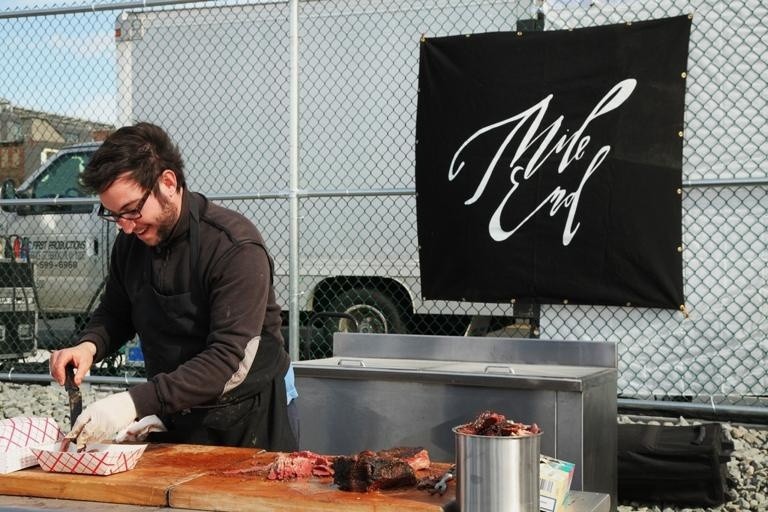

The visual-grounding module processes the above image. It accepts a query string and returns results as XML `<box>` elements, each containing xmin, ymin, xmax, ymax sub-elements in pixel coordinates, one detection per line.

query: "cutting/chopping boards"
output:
<box><xmin>168</xmin><ymin>449</ymin><xmax>458</xmax><ymax>512</ymax></box>
<box><xmin>1</xmin><ymin>439</ymin><xmax>269</xmax><ymax>507</ymax></box>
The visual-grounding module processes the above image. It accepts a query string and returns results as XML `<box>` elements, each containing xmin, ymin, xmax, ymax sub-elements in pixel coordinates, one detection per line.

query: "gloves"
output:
<box><xmin>63</xmin><ymin>390</ymin><xmax>139</xmax><ymax>453</ymax></box>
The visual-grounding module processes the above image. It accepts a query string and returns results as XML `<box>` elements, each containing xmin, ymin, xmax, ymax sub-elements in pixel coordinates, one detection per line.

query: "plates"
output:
<box><xmin>29</xmin><ymin>445</ymin><xmax>148</xmax><ymax>476</ymax></box>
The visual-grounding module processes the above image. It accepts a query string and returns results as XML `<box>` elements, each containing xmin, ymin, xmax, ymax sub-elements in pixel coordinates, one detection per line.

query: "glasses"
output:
<box><xmin>97</xmin><ymin>180</ymin><xmax>155</xmax><ymax>222</ymax></box>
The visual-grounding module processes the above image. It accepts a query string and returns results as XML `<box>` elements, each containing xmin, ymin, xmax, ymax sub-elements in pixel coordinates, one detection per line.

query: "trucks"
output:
<box><xmin>0</xmin><ymin>0</ymin><xmax>517</xmax><ymax>356</ymax></box>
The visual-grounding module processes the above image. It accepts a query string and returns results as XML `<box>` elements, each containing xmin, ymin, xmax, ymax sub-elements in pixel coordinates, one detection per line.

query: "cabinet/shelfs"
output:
<box><xmin>292</xmin><ymin>331</ymin><xmax>619</xmax><ymax>511</ymax></box>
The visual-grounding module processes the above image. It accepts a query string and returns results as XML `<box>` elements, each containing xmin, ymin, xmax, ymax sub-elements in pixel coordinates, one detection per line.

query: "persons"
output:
<box><xmin>48</xmin><ymin>123</ymin><xmax>300</xmax><ymax>453</ymax></box>
